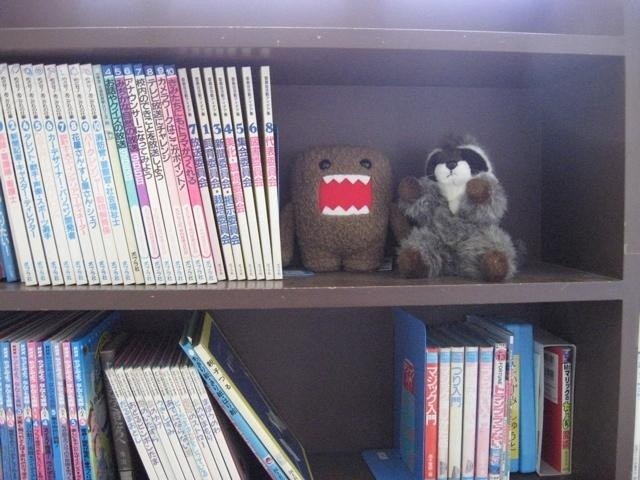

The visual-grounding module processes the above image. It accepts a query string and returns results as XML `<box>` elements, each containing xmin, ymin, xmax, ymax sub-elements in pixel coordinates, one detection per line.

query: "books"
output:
<box><xmin>401</xmin><ymin>314</ymin><xmax>577</xmax><ymax>480</ymax></box>
<box><xmin>1</xmin><ymin>311</ymin><xmax>313</xmax><ymax>480</ymax></box>
<box><xmin>1</xmin><ymin>62</ymin><xmax>283</xmax><ymax>289</ymax></box>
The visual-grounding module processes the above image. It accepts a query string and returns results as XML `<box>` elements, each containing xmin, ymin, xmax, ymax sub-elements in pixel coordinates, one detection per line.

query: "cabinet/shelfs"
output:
<box><xmin>0</xmin><ymin>0</ymin><xmax>640</xmax><ymax>480</ymax></box>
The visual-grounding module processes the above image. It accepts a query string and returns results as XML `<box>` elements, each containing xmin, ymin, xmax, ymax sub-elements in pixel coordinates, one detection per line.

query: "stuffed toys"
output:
<box><xmin>280</xmin><ymin>144</ymin><xmax>391</xmax><ymax>273</ymax></box>
<box><xmin>390</xmin><ymin>132</ymin><xmax>520</xmax><ymax>283</ymax></box>
